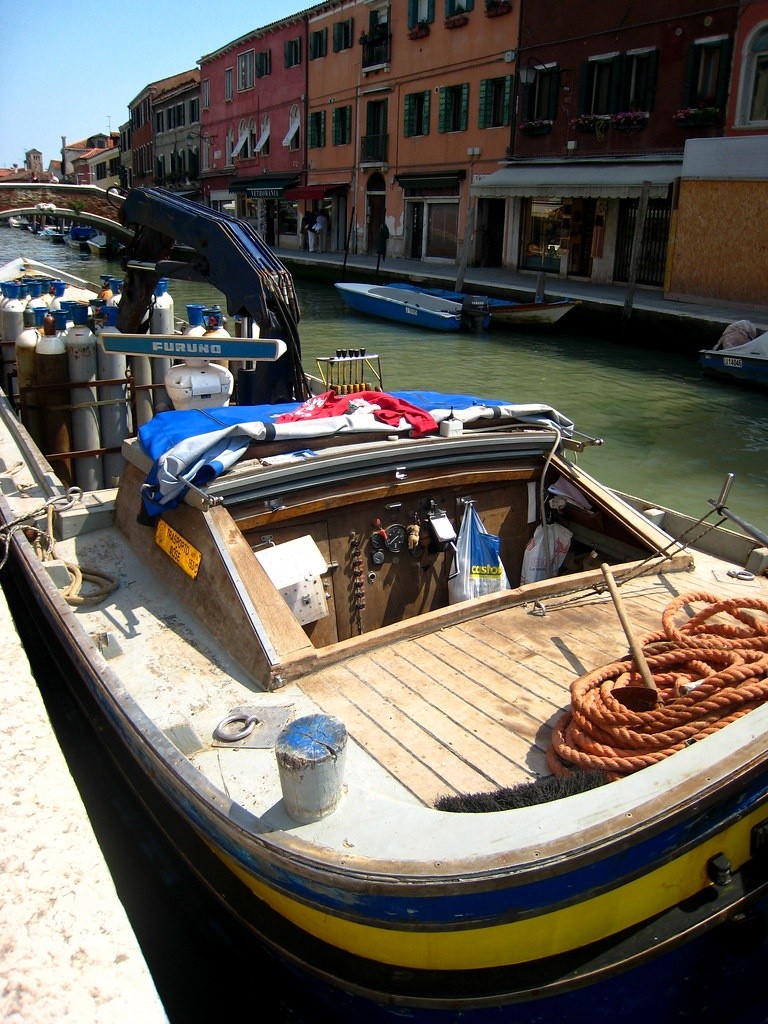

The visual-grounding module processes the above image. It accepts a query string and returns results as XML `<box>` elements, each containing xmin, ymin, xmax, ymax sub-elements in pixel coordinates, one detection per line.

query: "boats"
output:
<box><xmin>7</xmin><ymin>215</ymin><xmax>125</xmax><ymax>256</ymax></box>
<box><xmin>333</xmin><ymin>280</ymin><xmax>493</xmax><ymax>334</ymax></box>
<box><xmin>386</xmin><ymin>282</ymin><xmax>583</xmax><ymax>335</ymax></box>
<box><xmin>698</xmin><ymin>321</ymin><xmax>767</xmax><ymax>388</ymax></box>
<box><xmin>0</xmin><ymin>184</ymin><xmax>768</xmax><ymax>1024</ymax></box>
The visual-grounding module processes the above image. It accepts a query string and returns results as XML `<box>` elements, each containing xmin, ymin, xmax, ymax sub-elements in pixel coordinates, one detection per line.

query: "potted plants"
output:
<box><xmin>408</xmin><ymin>29</ymin><xmax>428</xmax><ymax>40</ymax></box>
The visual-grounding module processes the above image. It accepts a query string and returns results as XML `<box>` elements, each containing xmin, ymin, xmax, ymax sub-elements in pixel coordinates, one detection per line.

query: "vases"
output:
<box><xmin>485</xmin><ymin>7</ymin><xmax>511</xmax><ymax>17</ymax></box>
<box><xmin>443</xmin><ymin>17</ymin><xmax>470</xmax><ymax>29</ymax></box>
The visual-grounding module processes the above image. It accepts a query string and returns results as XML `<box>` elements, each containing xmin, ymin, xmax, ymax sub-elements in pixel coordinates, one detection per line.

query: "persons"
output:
<box><xmin>371</xmin><ymin>220</ymin><xmax>389</xmax><ymax>263</ymax></box>
<box><xmin>29</xmin><ymin>172</ymin><xmax>41</xmax><ymax>183</ymax></box>
<box><xmin>47</xmin><ymin>172</ymin><xmax>60</xmax><ymax>184</ymax></box>
<box><xmin>300</xmin><ymin>208</ymin><xmax>328</xmax><ymax>253</ymax></box>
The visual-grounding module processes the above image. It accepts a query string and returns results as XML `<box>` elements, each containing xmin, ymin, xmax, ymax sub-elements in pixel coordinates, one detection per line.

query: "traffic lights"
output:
<box><xmin>119</xmin><ymin>167</ymin><xmax>123</xmax><ymax>180</ymax></box>
<box><xmin>124</xmin><ymin>167</ymin><xmax>128</xmax><ymax>180</ymax></box>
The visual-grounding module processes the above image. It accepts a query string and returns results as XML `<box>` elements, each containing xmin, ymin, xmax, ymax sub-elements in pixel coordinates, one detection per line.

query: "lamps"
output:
<box><xmin>186</xmin><ymin>132</ymin><xmax>218</xmax><ymax>147</ymax></box>
<box><xmin>567</xmin><ymin>140</ymin><xmax>577</xmax><ymax>156</ymax></box>
<box><xmin>467</xmin><ymin>147</ymin><xmax>481</xmax><ymax>162</ymax></box>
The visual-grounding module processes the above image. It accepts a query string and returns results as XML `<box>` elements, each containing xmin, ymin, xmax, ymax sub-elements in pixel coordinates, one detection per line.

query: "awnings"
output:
<box><xmin>285</xmin><ymin>183</ymin><xmax>350</xmax><ymax>200</ymax></box>
<box><xmin>228</xmin><ymin>172</ymin><xmax>300</xmax><ymax>199</ymax></box>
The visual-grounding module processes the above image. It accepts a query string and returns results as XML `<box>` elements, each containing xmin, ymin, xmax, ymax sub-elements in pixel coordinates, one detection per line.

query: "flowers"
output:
<box><xmin>484</xmin><ymin>0</ymin><xmax>512</xmax><ymax>11</ymax></box>
<box><xmin>412</xmin><ymin>17</ymin><xmax>428</xmax><ymax>33</ymax></box>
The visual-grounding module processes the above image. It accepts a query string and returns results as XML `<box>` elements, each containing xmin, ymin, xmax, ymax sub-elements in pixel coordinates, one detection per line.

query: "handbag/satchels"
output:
<box><xmin>314</xmin><ymin>223</ymin><xmax>323</xmax><ymax>234</ymax></box>
<box><xmin>520</xmin><ymin>522</ymin><xmax>574</xmax><ymax>586</ymax></box>
<box><xmin>304</xmin><ymin>224</ymin><xmax>309</xmax><ymax>230</ymax></box>
<box><xmin>448</xmin><ymin>502</ymin><xmax>511</xmax><ymax>605</ymax></box>
